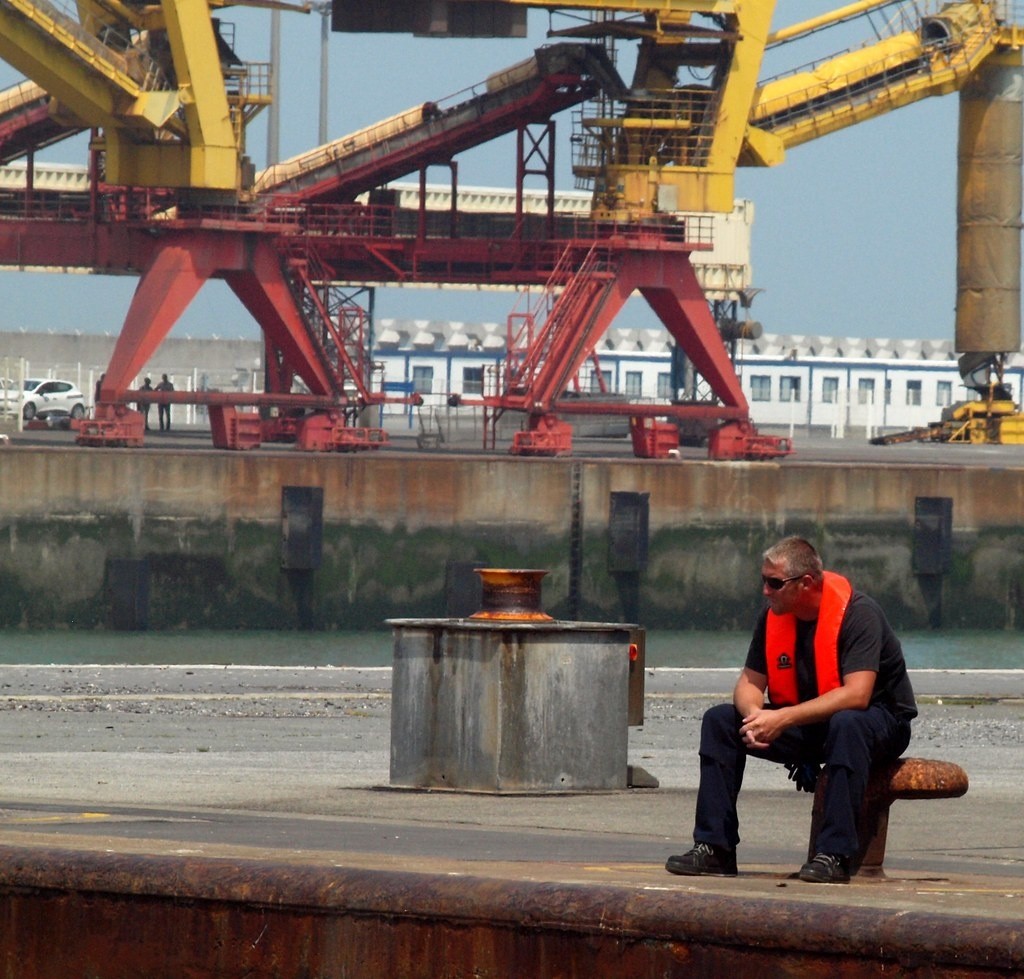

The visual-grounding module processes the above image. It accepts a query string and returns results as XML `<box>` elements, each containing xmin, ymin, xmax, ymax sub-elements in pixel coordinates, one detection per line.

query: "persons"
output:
<box><xmin>154</xmin><ymin>373</ymin><xmax>174</xmax><ymax>432</ymax></box>
<box><xmin>137</xmin><ymin>377</ymin><xmax>153</xmax><ymax>430</ymax></box>
<box><xmin>94</xmin><ymin>373</ymin><xmax>105</xmax><ymax>402</ymax></box>
<box><xmin>664</xmin><ymin>534</ymin><xmax>918</xmax><ymax>884</ymax></box>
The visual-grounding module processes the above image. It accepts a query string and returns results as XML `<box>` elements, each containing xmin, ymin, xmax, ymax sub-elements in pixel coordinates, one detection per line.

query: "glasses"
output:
<box><xmin>761</xmin><ymin>574</ymin><xmax>804</xmax><ymax>590</ymax></box>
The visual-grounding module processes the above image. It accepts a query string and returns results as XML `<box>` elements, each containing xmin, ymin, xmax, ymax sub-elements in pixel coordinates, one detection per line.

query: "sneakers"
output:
<box><xmin>665</xmin><ymin>842</ymin><xmax>738</xmax><ymax>877</ymax></box>
<box><xmin>799</xmin><ymin>852</ymin><xmax>851</xmax><ymax>884</ymax></box>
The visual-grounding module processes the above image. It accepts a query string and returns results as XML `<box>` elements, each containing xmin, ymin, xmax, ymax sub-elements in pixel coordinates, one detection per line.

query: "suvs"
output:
<box><xmin>0</xmin><ymin>378</ymin><xmax>89</xmax><ymax>422</ymax></box>
